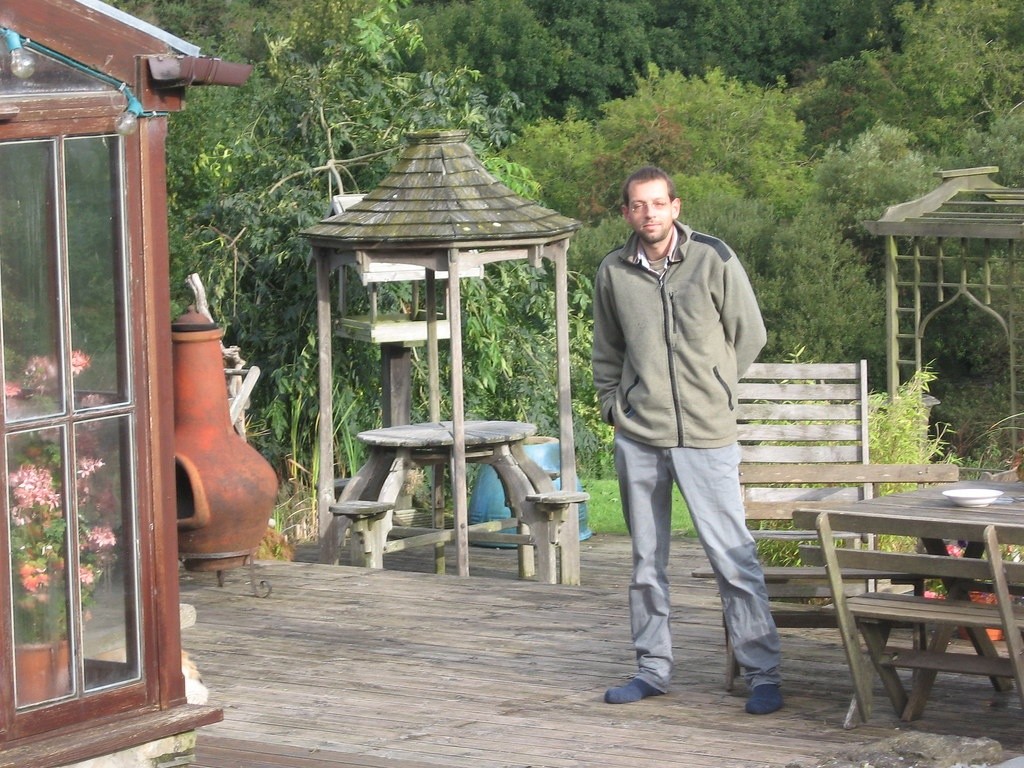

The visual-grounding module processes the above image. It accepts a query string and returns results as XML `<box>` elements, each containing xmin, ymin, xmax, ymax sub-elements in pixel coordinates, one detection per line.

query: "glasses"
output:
<box><xmin>629</xmin><ymin>199</ymin><xmax>669</xmax><ymax>213</ymax></box>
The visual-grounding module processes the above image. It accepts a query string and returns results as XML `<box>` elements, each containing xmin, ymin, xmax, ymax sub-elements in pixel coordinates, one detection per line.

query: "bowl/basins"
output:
<box><xmin>466</xmin><ymin>435</ymin><xmax>593</xmax><ymax>548</ymax></box>
<box><xmin>942</xmin><ymin>489</ymin><xmax>1005</xmax><ymax>508</ymax></box>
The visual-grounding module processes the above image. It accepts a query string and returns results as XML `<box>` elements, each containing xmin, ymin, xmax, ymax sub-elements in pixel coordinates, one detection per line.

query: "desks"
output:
<box><xmin>790</xmin><ymin>479</ymin><xmax>1024</xmax><ymax>722</ymax></box>
<box><xmin>338</xmin><ymin>420</ymin><xmax>557</xmax><ymax>577</ymax></box>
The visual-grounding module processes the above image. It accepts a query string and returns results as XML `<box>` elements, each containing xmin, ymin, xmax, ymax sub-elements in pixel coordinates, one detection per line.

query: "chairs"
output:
<box><xmin>690</xmin><ymin>359</ymin><xmax>1024</xmax><ymax>731</ymax></box>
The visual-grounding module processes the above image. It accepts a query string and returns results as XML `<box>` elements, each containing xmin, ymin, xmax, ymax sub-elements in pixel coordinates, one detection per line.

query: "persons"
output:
<box><xmin>592</xmin><ymin>164</ymin><xmax>785</xmax><ymax>716</ymax></box>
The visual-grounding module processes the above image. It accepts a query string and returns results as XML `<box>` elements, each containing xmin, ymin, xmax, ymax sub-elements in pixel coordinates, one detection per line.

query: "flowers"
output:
<box><xmin>5</xmin><ymin>340</ymin><xmax>122</xmax><ymax>644</ymax></box>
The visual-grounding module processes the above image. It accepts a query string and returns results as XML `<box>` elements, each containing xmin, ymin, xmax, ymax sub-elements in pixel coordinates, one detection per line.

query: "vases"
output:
<box><xmin>13</xmin><ymin>638</ymin><xmax>71</xmax><ymax>709</ymax></box>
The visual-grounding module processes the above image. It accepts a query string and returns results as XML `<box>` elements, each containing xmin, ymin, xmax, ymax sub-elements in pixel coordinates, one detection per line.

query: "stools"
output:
<box><xmin>309</xmin><ymin>469</ymin><xmax>591</xmax><ymax>585</ymax></box>
